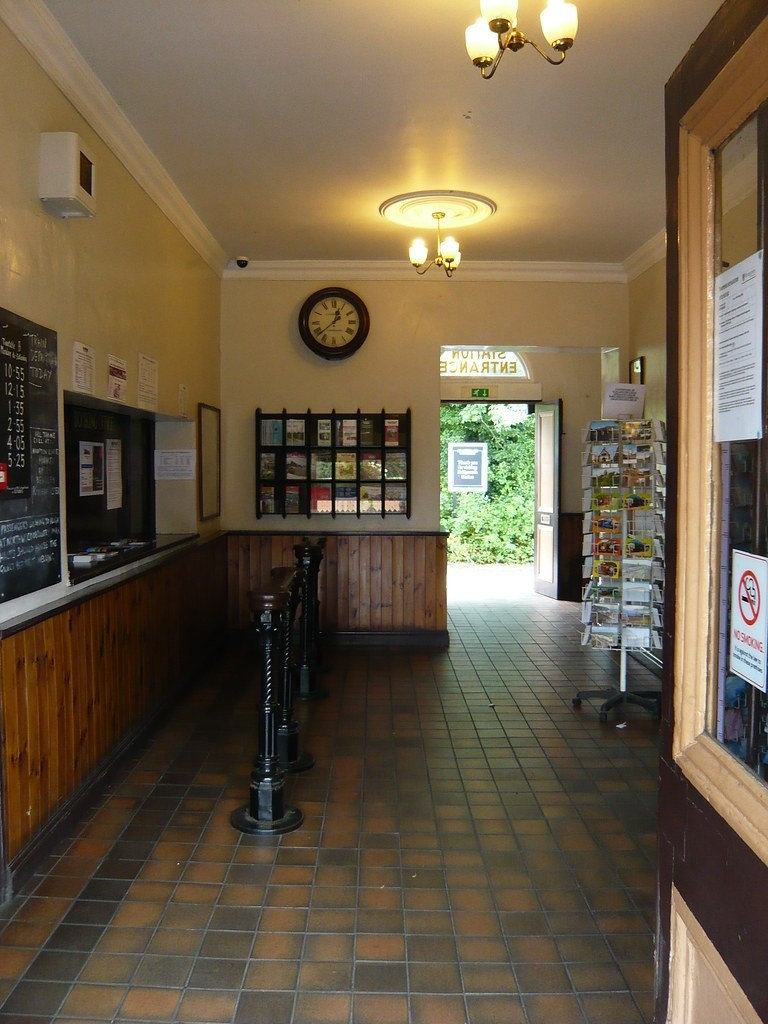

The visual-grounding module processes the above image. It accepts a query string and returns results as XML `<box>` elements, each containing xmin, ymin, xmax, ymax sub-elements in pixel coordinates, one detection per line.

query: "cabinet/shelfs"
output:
<box><xmin>254</xmin><ymin>407</ymin><xmax>412</xmax><ymax>520</ymax></box>
<box><xmin>572</xmin><ymin>413</ymin><xmax>667</xmax><ymax>722</ymax></box>
<box><xmin>722</xmin><ymin>443</ymin><xmax>768</xmax><ymax>781</ymax></box>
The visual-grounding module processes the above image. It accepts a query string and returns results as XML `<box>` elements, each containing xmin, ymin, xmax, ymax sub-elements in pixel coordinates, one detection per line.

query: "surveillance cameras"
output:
<box><xmin>237</xmin><ymin>256</ymin><xmax>249</xmax><ymax>268</ymax></box>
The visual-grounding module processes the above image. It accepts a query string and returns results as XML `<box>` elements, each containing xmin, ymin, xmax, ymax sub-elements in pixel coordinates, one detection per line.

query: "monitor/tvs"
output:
<box><xmin>62</xmin><ymin>389</ymin><xmax>158</xmax><ymax>555</ymax></box>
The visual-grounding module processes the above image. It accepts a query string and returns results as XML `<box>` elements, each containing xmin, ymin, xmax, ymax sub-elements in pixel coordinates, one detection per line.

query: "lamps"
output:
<box><xmin>465</xmin><ymin>0</ymin><xmax>579</xmax><ymax>80</ymax></box>
<box><xmin>379</xmin><ymin>191</ymin><xmax>496</xmax><ymax>278</ymax></box>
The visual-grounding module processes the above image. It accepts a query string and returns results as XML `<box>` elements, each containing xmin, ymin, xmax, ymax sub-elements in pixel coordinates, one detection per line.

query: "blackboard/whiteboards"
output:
<box><xmin>0</xmin><ymin>306</ymin><xmax>62</xmax><ymax>602</ymax></box>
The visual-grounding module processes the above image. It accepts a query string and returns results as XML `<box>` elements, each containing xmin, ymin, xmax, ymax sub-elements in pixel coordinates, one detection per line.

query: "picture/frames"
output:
<box><xmin>628</xmin><ymin>356</ymin><xmax>643</xmax><ymax>388</ymax></box>
<box><xmin>198</xmin><ymin>402</ymin><xmax>221</xmax><ymax>520</ymax></box>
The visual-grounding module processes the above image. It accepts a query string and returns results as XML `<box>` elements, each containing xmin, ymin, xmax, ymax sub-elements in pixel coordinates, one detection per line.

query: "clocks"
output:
<box><xmin>299</xmin><ymin>287</ymin><xmax>370</xmax><ymax>361</ymax></box>
